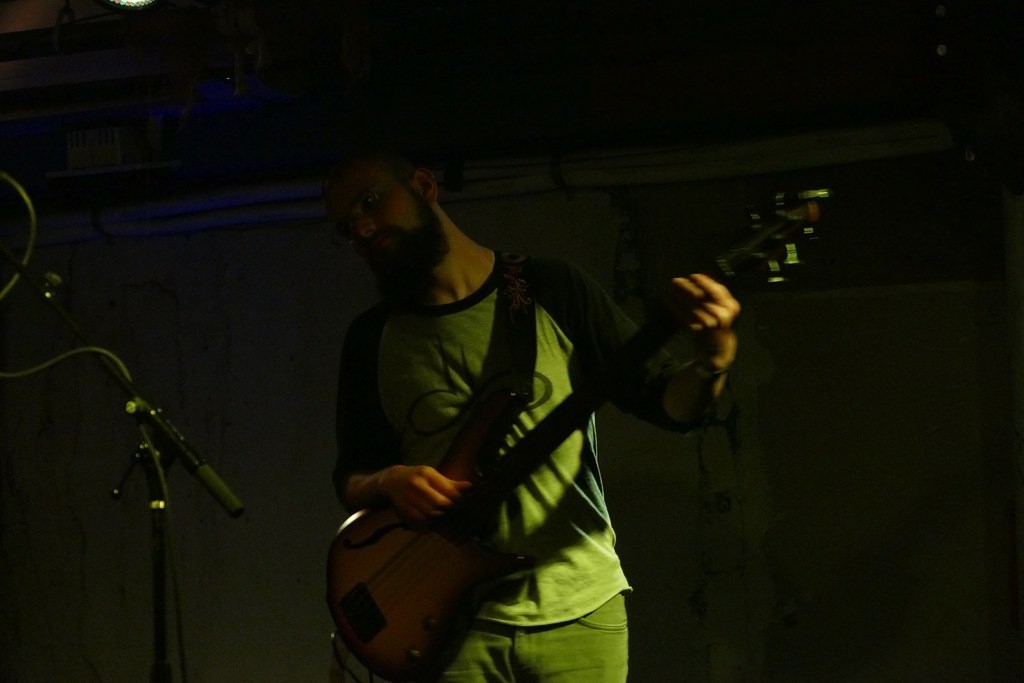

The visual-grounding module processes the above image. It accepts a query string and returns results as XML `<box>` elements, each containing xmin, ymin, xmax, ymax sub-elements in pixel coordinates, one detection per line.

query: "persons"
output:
<box><xmin>321</xmin><ymin>151</ymin><xmax>746</xmax><ymax>683</ymax></box>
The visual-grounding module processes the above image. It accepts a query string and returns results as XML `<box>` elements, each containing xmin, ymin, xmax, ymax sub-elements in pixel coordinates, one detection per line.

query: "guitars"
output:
<box><xmin>326</xmin><ymin>188</ymin><xmax>832</xmax><ymax>683</ymax></box>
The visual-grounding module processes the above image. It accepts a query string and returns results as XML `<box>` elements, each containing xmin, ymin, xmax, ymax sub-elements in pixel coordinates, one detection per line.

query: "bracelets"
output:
<box><xmin>694</xmin><ymin>365</ymin><xmax>729</xmax><ymax>381</ymax></box>
